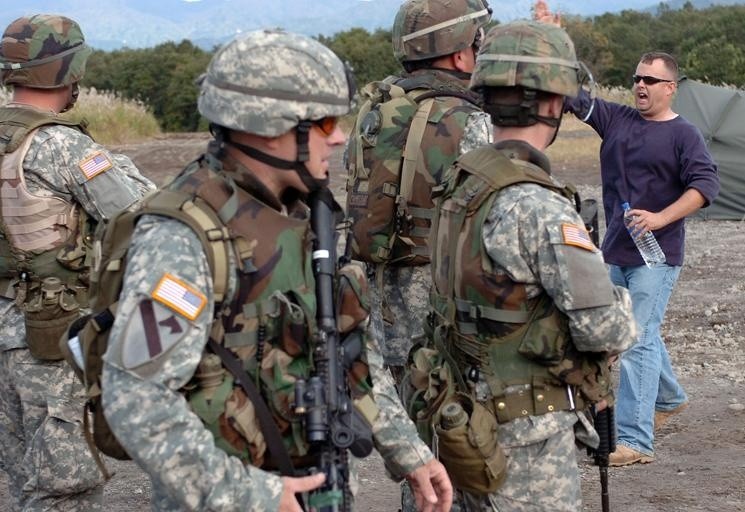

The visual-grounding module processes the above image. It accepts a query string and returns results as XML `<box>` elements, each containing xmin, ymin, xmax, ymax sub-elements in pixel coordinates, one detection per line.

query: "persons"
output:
<box><xmin>0</xmin><ymin>12</ymin><xmax>163</xmax><ymax>511</ymax></box>
<box><xmin>340</xmin><ymin>0</ymin><xmax>495</xmax><ymax>410</ymax></box>
<box><xmin>529</xmin><ymin>1</ymin><xmax>722</xmax><ymax>467</ymax></box>
<box><xmin>426</xmin><ymin>16</ymin><xmax>639</xmax><ymax>511</ymax></box>
<box><xmin>98</xmin><ymin>28</ymin><xmax>452</xmax><ymax>510</ymax></box>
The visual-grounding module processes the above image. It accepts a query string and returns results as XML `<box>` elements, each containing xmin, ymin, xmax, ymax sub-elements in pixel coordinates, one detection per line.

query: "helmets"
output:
<box><xmin>0</xmin><ymin>12</ymin><xmax>87</xmax><ymax>88</ymax></box>
<box><xmin>199</xmin><ymin>30</ymin><xmax>353</xmax><ymax>140</ymax></box>
<box><xmin>468</xmin><ymin>20</ymin><xmax>578</xmax><ymax>100</ymax></box>
<box><xmin>391</xmin><ymin>0</ymin><xmax>492</xmax><ymax>63</ymax></box>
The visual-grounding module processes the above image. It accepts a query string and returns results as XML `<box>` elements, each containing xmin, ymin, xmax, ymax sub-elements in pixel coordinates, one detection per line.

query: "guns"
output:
<box><xmin>293</xmin><ymin>189</ymin><xmax>372</xmax><ymax>512</ymax></box>
<box><xmin>560</xmin><ymin>200</ymin><xmax>617</xmax><ymax>511</ymax></box>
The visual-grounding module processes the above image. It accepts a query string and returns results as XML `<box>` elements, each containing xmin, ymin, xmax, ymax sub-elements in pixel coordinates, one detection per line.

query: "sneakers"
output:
<box><xmin>655</xmin><ymin>400</ymin><xmax>688</xmax><ymax>429</ymax></box>
<box><xmin>609</xmin><ymin>443</ymin><xmax>653</xmax><ymax>466</ymax></box>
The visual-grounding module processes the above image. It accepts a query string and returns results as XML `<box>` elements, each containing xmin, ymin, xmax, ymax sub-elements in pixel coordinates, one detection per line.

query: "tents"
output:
<box><xmin>669</xmin><ymin>72</ymin><xmax>745</xmax><ymax>223</ymax></box>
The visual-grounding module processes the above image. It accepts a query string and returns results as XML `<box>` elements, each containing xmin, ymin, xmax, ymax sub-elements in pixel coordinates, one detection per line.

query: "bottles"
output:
<box><xmin>440</xmin><ymin>404</ymin><xmax>505</xmax><ymax>496</ymax></box>
<box><xmin>621</xmin><ymin>203</ymin><xmax>667</xmax><ymax>269</ymax></box>
<box><xmin>24</xmin><ymin>277</ymin><xmax>82</xmax><ymax>361</ymax></box>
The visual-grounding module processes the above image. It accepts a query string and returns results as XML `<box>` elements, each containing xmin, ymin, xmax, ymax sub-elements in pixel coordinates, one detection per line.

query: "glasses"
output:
<box><xmin>632</xmin><ymin>73</ymin><xmax>671</xmax><ymax>87</ymax></box>
<box><xmin>314</xmin><ymin>113</ymin><xmax>339</xmax><ymax>135</ymax></box>
